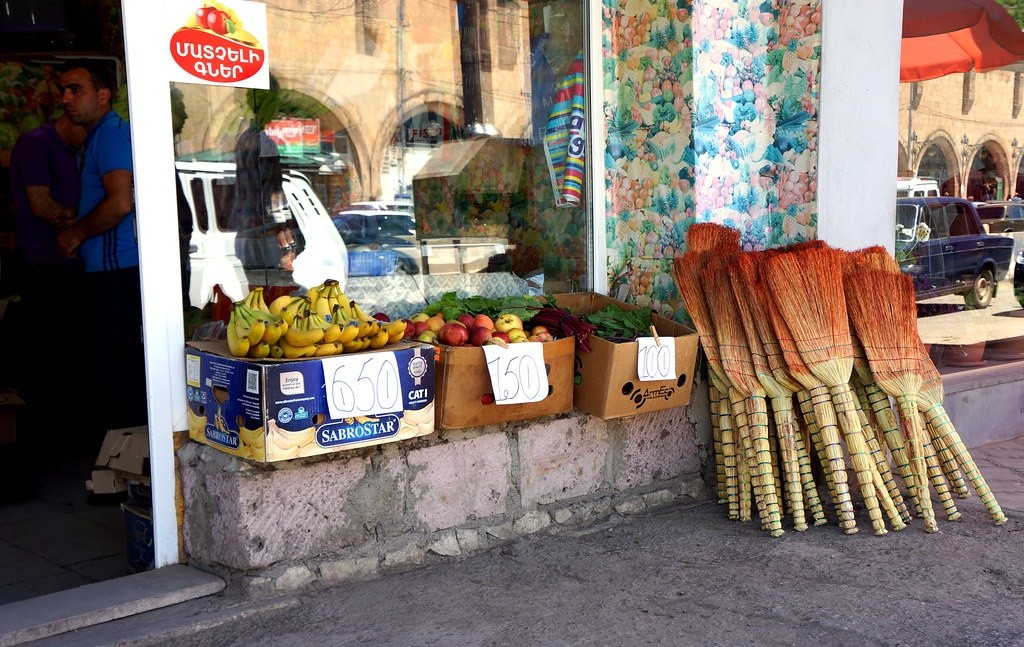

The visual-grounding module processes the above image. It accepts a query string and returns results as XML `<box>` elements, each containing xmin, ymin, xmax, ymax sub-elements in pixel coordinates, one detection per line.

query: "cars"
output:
<box><xmin>332</xmin><ymin>202</ymin><xmax>420</xmax><ymax>277</ymax></box>
<box><xmin>176</xmin><ymin>159</ymin><xmax>348</xmax><ymax>312</ymax></box>
<box><xmin>895</xmin><ymin>198</ymin><xmax>1014</xmax><ymax>309</ymax></box>
<box><xmin>896</xmin><ymin>178</ymin><xmax>1024</xmax><ymax>233</ymax></box>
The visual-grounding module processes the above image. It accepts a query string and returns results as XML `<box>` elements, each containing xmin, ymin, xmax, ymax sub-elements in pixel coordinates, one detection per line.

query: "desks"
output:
<box><xmin>343</xmin><ymin>272</ymin><xmax>530</xmax><ymax>324</ymax></box>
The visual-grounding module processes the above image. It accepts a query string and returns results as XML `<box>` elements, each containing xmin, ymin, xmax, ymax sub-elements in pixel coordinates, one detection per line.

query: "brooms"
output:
<box><xmin>668</xmin><ymin>222</ymin><xmax>1010</xmax><ymax>539</ymax></box>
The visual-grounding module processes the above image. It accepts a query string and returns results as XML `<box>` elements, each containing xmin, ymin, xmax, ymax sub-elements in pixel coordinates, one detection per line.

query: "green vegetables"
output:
<box><xmin>423</xmin><ymin>290</ymin><xmax>658</xmax><ymax>353</ymax></box>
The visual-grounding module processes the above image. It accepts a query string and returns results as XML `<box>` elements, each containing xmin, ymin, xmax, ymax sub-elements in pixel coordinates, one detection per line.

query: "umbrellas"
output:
<box><xmin>899</xmin><ymin>0</ymin><xmax>1024</xmax><ymax>86</ymax></box>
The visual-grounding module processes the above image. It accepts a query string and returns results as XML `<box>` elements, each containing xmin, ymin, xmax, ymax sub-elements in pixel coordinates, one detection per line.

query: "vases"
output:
<box><xmin>923</xmin><ymin>309</ymin><xmax>1024</xmax><ymax>361</ymax></box>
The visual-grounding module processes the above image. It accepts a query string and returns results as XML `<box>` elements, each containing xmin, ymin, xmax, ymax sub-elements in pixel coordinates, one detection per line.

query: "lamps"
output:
<box><xmin>911</xmin><ymin>130</ymin><xmax>937</xmax><ymax>157</ymax></box>
<box><xmin>961</xmin><ymin>135</ymin><xmax>987</xmax><ymax>162</ymax></box>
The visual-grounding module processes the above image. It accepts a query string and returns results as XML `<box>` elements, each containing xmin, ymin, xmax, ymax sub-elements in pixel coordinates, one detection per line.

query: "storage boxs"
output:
<box><xmin>532</xmin><ymin>291</ymin><xmax>699</xmax><ymax>421</ymax></box>
<box><xmin>0</xmin><ymin>386</ymin><xmax>26</xmax><ymax>445</ymax></box>
<box><xmin>178</xmin><ymin>337</ymin><xmax>437</xmax><ymax>461</ymax></box>
<box><xmin>435</xmin><ymin>331</ymin><xmax>574</xmax><ymax>432</ymax></box>
<box><xmin>348</xmin><ymin>249</ymin><xmax>398</xmax><ymax>276</ymax></box>
<box><xmin>87</xmin><ymin>426</ymin><xmax>155</xmax><ymax>572</ymax></box>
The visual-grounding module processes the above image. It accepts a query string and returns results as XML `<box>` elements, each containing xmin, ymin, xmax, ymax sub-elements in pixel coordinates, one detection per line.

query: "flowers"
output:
<box><xmin>893</xmin><ymin>222</ymin><xmax>931</xmax><ymax>265</ymax></box>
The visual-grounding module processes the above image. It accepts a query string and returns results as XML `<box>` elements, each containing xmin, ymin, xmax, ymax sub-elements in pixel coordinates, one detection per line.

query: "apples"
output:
<box><xmin>371</xmin><ymin>312</ymin><xmax>553</xmax><ymax>346</ymax></box>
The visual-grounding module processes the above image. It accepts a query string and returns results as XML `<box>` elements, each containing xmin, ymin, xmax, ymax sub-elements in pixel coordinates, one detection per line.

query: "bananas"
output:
<box><xmin>227</xmin><ymin>278</ymin><xmax>407</xmax><ymax>358</ymax></box>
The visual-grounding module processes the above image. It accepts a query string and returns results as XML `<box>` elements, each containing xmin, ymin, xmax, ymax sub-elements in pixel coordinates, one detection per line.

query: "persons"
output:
<box><xmin>234</xmin><ymin>73</ymin><xmax>306</xmax><ymax>295</ymax></box>
<box><xmin>170</xmin><ymin>86</ymin><xmax>193</xmax><ymax>323</ymax></box>
<box><xmin>10</xmin><ymin>112</ymin><xmax>88</xmax><ymax>360</ymax></box>
<box><xmin>968</xmin><ymin>184</ymin><xmax>996</xmax><ymax>202</ymax></box>
<box><xmin>55</xmin><ymin>58</ymin><xmax>143</xmax><ymax>430</ymax></box>
<box><xmin>1012</xmin><ymin>193</ymin><xmax>1022</xmax><ymax>201</ymax></box>
